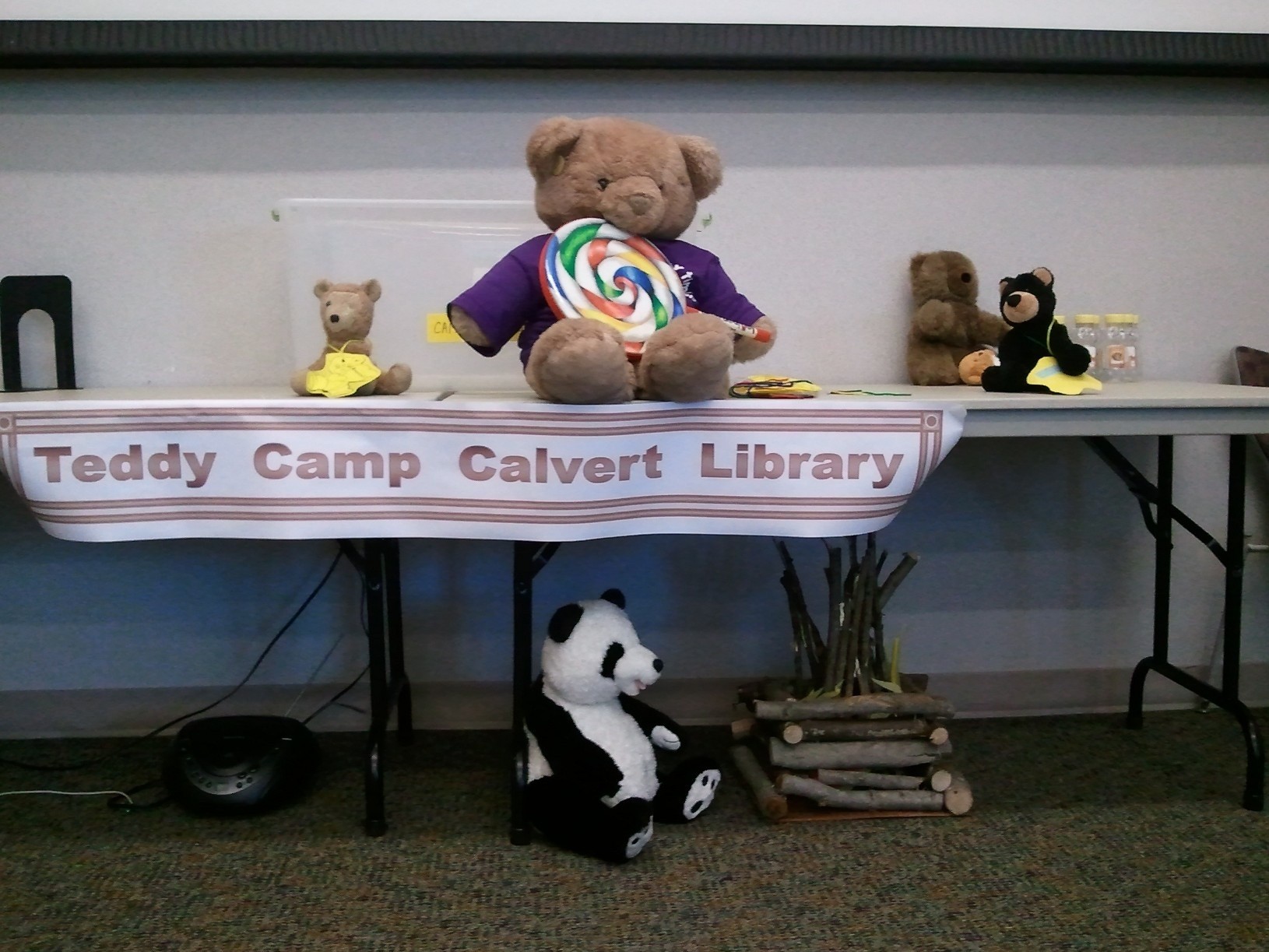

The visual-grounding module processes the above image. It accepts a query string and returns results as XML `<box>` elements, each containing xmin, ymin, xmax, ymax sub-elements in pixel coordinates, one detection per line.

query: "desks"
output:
<box><xmin>0</xmin><ymin>377</ymin><xmax>1269</xmax><ymax>835</ymax></box>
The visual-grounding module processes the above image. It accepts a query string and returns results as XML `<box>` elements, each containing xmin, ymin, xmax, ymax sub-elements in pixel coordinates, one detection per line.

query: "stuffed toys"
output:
<box><xmin>980</xmin><ymin>266</ymin><xmax>1092</xmax><ymax>393</ymax></box>
<box><xmin>445</xmin><ymin>116</ymin><xmax>777</xmax><ymax>405</ymax></box>
<box><xmin>289</xmin><ymin>278</ymin><xmax>411</xmax><ymax>399</ymax></box>
<box><xmin>905</xmin><ymin>251</ymin><xmax>1012</xmax><ymax>386</ymax></box>
<box><xmin>521</xmin><ymin>588</ymin><xmax>721</xmax><ymax>865</ymax></box>
<box><xmin>958</xmin><ymin>349</ymin><xmax>995</xmax><ymax>386</ymax></box>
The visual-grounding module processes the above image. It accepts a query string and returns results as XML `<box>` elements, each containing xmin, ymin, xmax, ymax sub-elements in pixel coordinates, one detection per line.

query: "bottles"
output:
<box><xmin>1095</xmin><ymin>314</ymin><xmax>1142</xmax><ymax>384</ymax></box>
<box><xmin>1072</xmin><ymin>314</ymin><xmax>1107</xmax><ymax>381</ymax></box>
<box><xmin>1054</xmin><ymin>315</ymin><xmax>1067</xmax><ymax>325</ymax></box>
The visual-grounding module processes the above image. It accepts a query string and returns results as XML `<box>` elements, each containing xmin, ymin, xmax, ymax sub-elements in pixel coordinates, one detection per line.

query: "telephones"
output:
<box><xmin>163</xmin><ymin>713</ymin><xmax>318</xmax><ymax>816</ymax></box>
